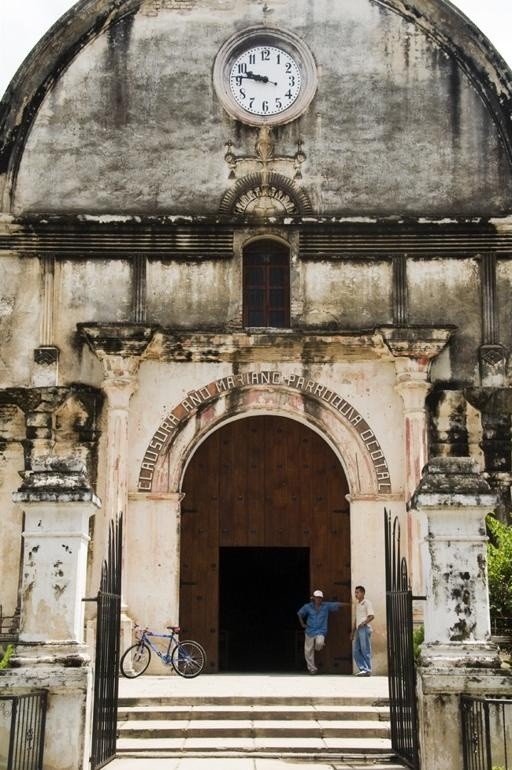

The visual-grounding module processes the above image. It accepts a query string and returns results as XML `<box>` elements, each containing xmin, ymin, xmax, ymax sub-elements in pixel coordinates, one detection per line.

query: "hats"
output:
<box><xmin>313</xmin><ymin>589</ymin><xmax>323</xmax><ymax>598</ymax></box>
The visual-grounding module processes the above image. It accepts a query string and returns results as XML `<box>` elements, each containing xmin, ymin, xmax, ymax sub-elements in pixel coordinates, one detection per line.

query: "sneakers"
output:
<box><xmin>310</xmin><ymin>670</ymin><xmax>317</xmax><ymax>676</ymax></box>
<box><xmin>355</xmin><ymin>671</ymin><xmax>372</xmax><ymax>677</ymax></box>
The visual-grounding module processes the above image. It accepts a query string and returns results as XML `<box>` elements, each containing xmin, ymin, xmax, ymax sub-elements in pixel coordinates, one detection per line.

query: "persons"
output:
<box><xmin>349</xmin><ymin>586</ymin><xmax>375</xmax><ymax>677</ymax></box>
<box><xmin>296</xmin><ymin>589</ymin><xmax>351</xmax><ymax>675</ymax></box>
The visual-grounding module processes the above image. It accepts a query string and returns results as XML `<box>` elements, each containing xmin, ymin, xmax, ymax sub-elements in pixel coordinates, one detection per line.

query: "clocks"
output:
<box><xmin>211</xmin><ymin>24</ymin><xmax>318</xmax><ymax>128</ymax></box>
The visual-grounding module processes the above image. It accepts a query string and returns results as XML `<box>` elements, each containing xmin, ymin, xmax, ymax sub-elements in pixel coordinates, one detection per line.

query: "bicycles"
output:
<box><xmin>119</xmin><ymin>620</ymin><xmax>208</xmax><ymax>680</ymax></box>
